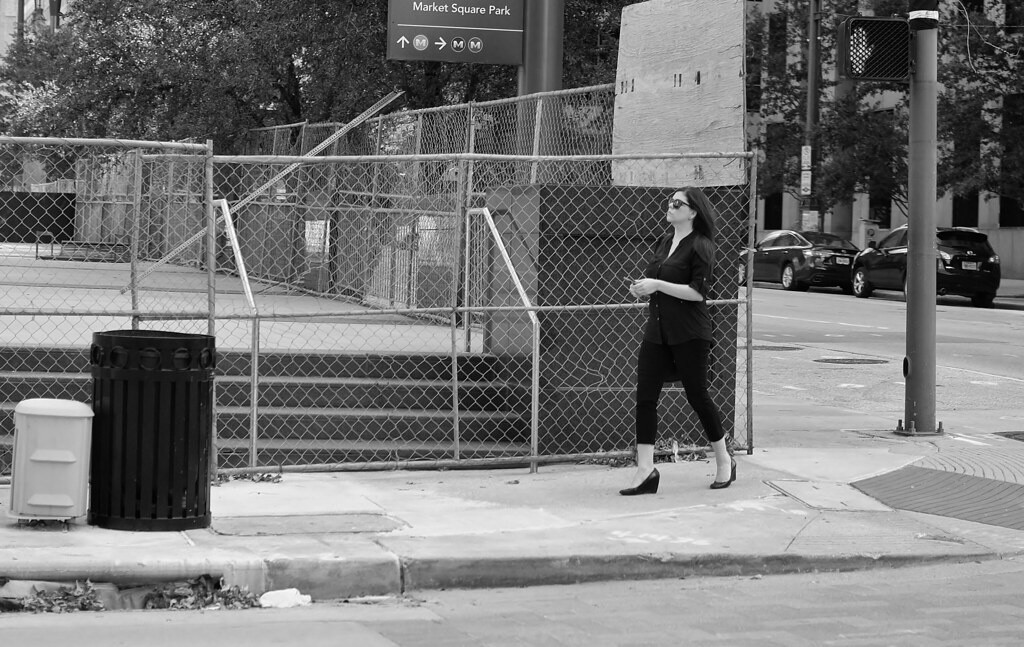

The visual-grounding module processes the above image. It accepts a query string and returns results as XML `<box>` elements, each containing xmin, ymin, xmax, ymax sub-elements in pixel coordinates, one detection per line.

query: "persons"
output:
<box><xmin>619</xmin><ymin>187</ymin><xmax>737</xmax><ymax>496</ymax></box>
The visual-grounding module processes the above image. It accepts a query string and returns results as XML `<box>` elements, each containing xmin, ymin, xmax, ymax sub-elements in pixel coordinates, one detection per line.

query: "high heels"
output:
<box><xmin>710</xmin><ymin>457</ymin><xmax>736</xmax><ymax>489</ymax></box>
<box><xmin>619</xmin><ymin>467</ymin><xmax>660</xmax><ymax>495</ymax></box>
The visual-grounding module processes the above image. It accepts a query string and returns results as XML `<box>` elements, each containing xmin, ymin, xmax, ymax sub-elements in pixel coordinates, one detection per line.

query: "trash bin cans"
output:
<box><xmin>6</xmin><ymin>397</ymin><xmax>94</xmax><ymax>526</ymax></box>
<box><xmin>88</xmin><ymin>329</ymin><xmax>218</xmax><ymax>534</ymax></box>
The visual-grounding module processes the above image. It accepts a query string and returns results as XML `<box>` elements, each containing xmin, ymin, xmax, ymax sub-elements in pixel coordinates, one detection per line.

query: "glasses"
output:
<box><xmin>669</xmin><ymin>197</ymin><xmax>689</xmax><ymax>209</ymax></box>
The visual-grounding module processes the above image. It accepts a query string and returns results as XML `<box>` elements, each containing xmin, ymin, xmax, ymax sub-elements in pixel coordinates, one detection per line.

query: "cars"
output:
<box><xmin>737</xmin><ymin>230</ymin><xmax>859</xmax><ymax>290</ymax></box>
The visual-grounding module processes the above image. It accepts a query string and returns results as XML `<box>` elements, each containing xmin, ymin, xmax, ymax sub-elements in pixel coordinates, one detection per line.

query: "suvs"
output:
<box><xmin>851</xmin><ymin>223</ymin><xmax>1001</xmax><ymax>303</ymax></box>
